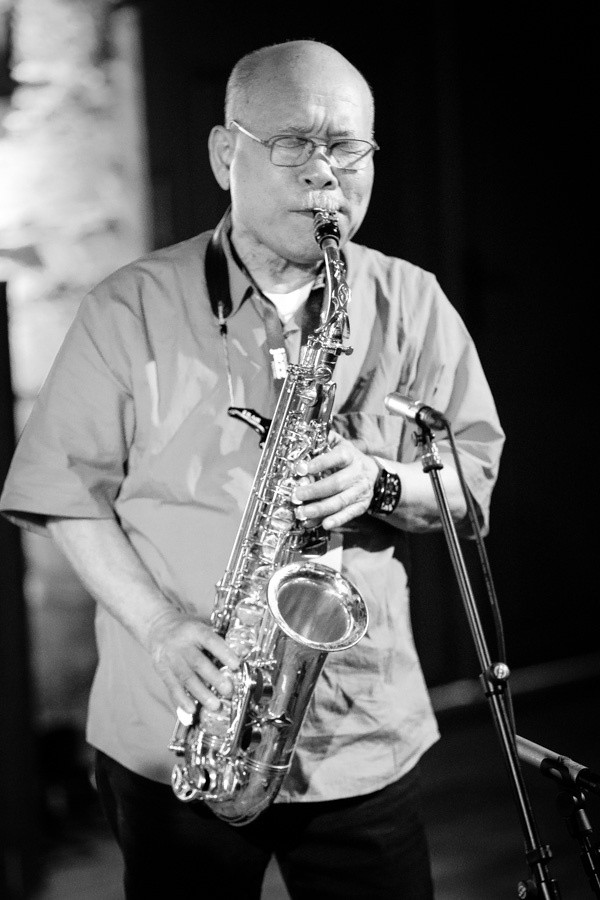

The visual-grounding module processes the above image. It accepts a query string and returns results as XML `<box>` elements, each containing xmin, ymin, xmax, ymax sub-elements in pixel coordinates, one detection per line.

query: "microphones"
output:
<box><xmin>515</xmin><ymin>735</ymin><xmax>600</xmax><ymax>796</ymax></box>
<box><xmin>382</xmin><ymin>391</ymin><xmax>451</xmax><ymax>431</ymax></box>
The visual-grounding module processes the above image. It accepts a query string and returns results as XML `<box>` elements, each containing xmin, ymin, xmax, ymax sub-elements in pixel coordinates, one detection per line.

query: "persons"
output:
<box><xmin>0</xmin><ymin>40</ymin><xmax>505</xmax><ymax>900</ymax></box>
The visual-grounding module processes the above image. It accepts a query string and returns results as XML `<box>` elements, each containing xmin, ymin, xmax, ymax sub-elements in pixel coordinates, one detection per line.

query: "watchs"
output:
<box><xmin>368</xmin><ymin>455</ymin><xmax>402</xmax><ymax>517</ymax></box>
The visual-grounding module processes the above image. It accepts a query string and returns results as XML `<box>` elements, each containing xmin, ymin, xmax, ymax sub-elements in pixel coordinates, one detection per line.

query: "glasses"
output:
<box><xmin>228</xmin><ymin>120</ymin><xmax>380</xmax><ymax>170</ymax></box>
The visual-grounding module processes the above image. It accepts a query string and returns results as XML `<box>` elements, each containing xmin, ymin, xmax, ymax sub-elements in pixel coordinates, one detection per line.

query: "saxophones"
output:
<box><xmin>166</xmin><ymin>203</ymin><xmax>372</xmax><ymax>833</ymax></box>
